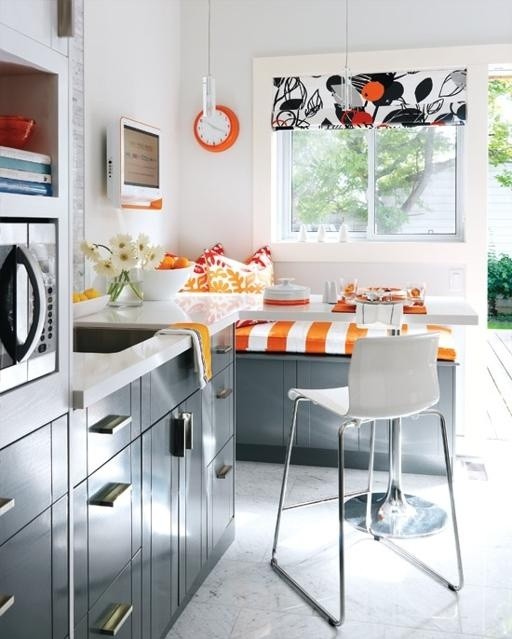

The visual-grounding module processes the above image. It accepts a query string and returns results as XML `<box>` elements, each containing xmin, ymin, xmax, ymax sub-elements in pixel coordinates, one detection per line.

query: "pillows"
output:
<box><xmin>173</xmin><ymin>243</ymin><xmax>279</xmax><ymax>331</ymax></box>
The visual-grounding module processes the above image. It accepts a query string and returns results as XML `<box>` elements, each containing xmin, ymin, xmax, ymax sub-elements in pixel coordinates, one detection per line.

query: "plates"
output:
<box><xmin>73</xmin><ymin>294</ymin><xmax>111</xmax><ymax>320</ymax></box>
<box><xmin>354</xmin><ymin>286</ymin><xmax>407</xmax><ymax>304</ymax></box>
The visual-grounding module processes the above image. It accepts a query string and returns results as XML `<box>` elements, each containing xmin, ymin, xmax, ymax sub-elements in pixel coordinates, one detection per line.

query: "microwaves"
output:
<box><xmin>0</xmin><ymin>223</ymin><xmax>58</xmax><ymax>391</ymax></box>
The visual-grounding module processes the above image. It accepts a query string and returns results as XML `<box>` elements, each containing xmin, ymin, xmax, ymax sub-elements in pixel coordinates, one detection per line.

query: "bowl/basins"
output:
<box><xmin>0</xmin><ymin>117</ymin><xmax>37</xmax><ymax>150</ymax></box>
<box><xmin>133</xmin><ymin>262</ymin><xmax>197</xmax><ymax>299</ymax></box>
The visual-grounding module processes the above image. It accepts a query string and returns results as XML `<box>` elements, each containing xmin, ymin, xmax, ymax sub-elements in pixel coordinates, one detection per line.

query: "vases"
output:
<box><xmin>105</xmin><ymin>266</ymin><xmax>145</xmax><ymax>309</ymax></box>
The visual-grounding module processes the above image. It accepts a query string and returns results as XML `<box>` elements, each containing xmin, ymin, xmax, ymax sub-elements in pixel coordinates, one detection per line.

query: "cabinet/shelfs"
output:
<box><xmin>203</xmin><ymin>326</ymin><xmax>237</xmax><ymax>579</ymax></box>
<box><xmin>0</xmin><ymin>412</ymin><xmax>71</xmax><ymax>639</ymax></box>
<box><xmin>71</xmin><ymin>378</ymin><xmax>143</xmax><ymax>639</ymax></box>
<box><xmin>142</xmin><ymin>350</ymin><xmax>204</xmax><ymax>637</ymax></box>
<box><xmin>0</xmin><ymin>1</ymin><xmax>75</xmax><ymax>450</ymax></box>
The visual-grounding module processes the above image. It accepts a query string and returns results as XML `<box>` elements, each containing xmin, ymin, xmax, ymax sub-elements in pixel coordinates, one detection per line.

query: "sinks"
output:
<box><xmin>72</xmin><ymin>318</ymin><xmax>169</xmax><ymax>356</ymax></box>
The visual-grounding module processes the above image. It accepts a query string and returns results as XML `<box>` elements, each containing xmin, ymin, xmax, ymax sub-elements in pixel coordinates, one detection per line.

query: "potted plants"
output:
<box><xmin>488</xmin><ymin>252</ymin><xmax>512</xmax><ymax>321</ymax></box>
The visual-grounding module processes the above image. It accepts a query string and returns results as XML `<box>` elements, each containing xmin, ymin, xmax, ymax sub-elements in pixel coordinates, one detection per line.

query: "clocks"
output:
<box><xmin>193</xmin><ymin>105</ymin><xmax>240</xmax><ymax>153</ymax></box>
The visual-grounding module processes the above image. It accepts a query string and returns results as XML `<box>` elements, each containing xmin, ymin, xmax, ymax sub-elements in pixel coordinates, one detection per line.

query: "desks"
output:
<box><xmin>213</xmin><ymin>321</ymin><xmax>461</xmax><ymax>479</ymax></box>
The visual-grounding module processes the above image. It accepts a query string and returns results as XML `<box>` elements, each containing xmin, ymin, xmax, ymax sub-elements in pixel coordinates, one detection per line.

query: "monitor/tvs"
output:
<box><xmin>106</xmin><ymin>116</ymin><xmax>164</xmax><ymax>199</ymax></box>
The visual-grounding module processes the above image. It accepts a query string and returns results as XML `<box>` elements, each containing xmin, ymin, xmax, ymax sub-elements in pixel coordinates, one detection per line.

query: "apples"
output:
<box><xmin>351</xmin><ymin>112</ymin><xmax>372</xmax><ymax>124</ymax></box>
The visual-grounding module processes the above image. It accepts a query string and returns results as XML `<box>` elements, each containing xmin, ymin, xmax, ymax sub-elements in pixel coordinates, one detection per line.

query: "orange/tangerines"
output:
<box><xmin>73</xmin><ymin>289</ymin><xmax>98</xmax><ymax>303</ymax></box>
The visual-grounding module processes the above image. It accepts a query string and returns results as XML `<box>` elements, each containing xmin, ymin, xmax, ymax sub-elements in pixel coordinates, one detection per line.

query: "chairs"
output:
<box><xmin>268</xmin><ymin>331</ymin><xmax>465</xmax><ymax>627</ymax></box>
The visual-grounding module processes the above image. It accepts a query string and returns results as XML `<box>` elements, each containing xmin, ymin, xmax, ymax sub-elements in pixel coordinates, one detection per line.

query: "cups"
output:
<box><xmin>342</xmin><ymin>278</ymin><xmax>358</xmax><ymax>302</ymax></box>
<box><xmin>406</xmin><ymin>281</ymin><xmax>424</xmax><ymax>301</ymax></box>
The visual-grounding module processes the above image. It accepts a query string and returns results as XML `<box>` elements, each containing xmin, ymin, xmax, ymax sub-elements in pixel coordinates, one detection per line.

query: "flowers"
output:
<box><xmin>78</xmin><ymin>232</ymin><xmax>164</xmax><ymax>303</ymax></box>
